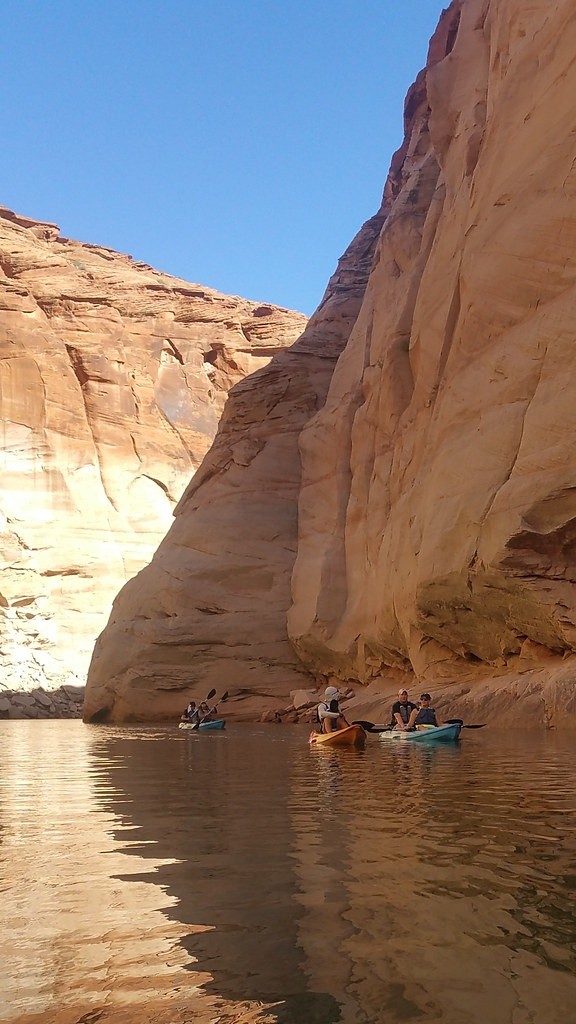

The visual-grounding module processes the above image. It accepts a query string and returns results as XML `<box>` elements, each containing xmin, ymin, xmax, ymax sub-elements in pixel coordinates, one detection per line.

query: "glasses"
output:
<box><xmin>420</xmin><ymin>698</ymin><xmax>427</xmax><ymax>701</ymax></box>
<box><xmin>332</xmin><ymin>689</ymin><xmax>339</xmax><ymax>695</ymax></box>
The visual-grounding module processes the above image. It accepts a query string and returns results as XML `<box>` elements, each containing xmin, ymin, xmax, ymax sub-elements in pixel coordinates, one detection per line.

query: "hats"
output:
<box><xmin>398</xmin><ymin>688</ymin><xmax>407</xmax><ymax>695</ymax></box>
<box><xmin>324</xmin><ymin>687</ymin><xmax>341</xmax><ymax>701</ymax></box>
<box><xmin>421</xmin><ymin>693</ymin><xmax>430</xmax><ymax>700</ymax></box>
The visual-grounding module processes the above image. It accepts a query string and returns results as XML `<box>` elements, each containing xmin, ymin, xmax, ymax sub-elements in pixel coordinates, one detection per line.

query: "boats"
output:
<box><xmin>379</xmin><ymin>722</ymin><xmax>464</xmax><ymax>745</ymax></box>
<box><xmin>179</xmin><ymin>720</ymin><xmax>227</xmax><ymax>731</ymax></box>
<box><xmin>308</xmin><ymin>723</ymin><xmax>367</xmax><ymax>747</ymax></box>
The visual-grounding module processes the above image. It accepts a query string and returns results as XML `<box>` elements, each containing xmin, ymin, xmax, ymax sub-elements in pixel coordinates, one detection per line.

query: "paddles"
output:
<box><xmin>350</xmin><ymin>717</ymin><xmax>488</xmax><ymax>734</ymax></box>
<box><xmin>181</xmin><ymin>687</ymin><xmax>218</xmax><ymax>729</ymax></box>
<box><xmin>190</xmin><ymin>691</ymin><xmax>228</xmax><ymax>730</ymax></box>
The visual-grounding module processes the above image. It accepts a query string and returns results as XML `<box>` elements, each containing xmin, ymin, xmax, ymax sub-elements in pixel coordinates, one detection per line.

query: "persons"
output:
<box><xmin>404</xmin><ymin>694</ymin><xmax>439</xmax><ymax>732</ymax></box>
<box><xmin>181</xmin><ymin>702</ymin><xmax>198</xmax><ymax>724</ymax></box>
<box><xmin>198</xmin><ymin>701</ymin><xmax>217</xmax><ymax>724</ymax></box>
<box><xmin>390</xmin><ymin>688</ymin><xmax>417</xmax><ymax>732</ymax></box>
<box><xmin>317</xmin><ymin>687</ymin><xmax>349</xmax><ymax>734</ymax></box>
